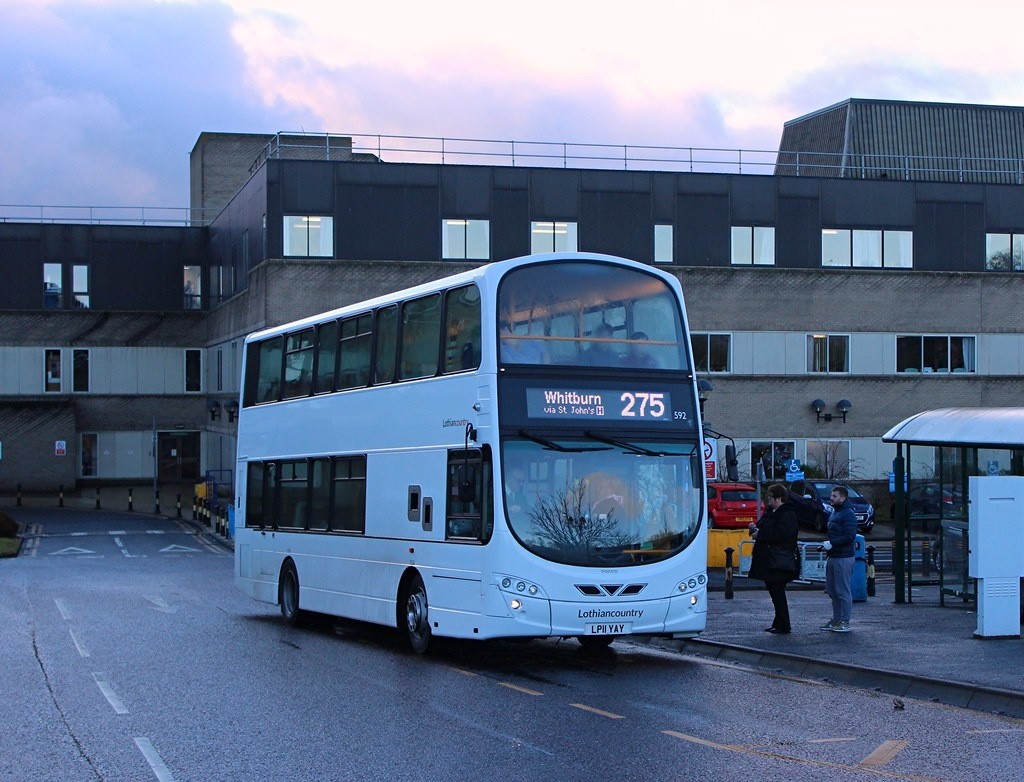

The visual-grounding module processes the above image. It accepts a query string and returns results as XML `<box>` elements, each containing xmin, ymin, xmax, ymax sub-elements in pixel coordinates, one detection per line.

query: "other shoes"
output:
<box><xmin>765</xmin><ymin>626</ymin><xmax>773</xmax><ymax>632</ymax></box>
<box><xmin>770</xmin><ymin>627</ymin><xmax>790</xmax><ymax>633</ymax></box>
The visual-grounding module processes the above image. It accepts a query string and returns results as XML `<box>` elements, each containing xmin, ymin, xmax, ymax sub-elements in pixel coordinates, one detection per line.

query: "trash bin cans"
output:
<box><xmin>227</xmin><ymin>502</ymin><xmax>235</xmax><ymax>540</ymax></box>
<box><xmin>850</xmin><ymin>534</ymin><xmax>868</xmax><ymax>602</ymax></box>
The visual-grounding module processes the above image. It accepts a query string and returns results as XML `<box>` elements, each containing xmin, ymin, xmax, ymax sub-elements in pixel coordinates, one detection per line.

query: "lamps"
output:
<box><xmin>829</xmin><ymin>399</ymin><xmax>852</xmax><ymax>423</ymax></box>
<box><xmin>206</xmin><ymin>400</ymin><xmax>221</xmax><ymax>421</ymax></box>
<box><xmin>811</xmin><ymin>398</ymin><xmax>828</xmax><ymax>424</ymax></box>
<box><xmin>224</xmin><ymin>399</ymin><xmax>239</xmax><ymax>423</ymax></box>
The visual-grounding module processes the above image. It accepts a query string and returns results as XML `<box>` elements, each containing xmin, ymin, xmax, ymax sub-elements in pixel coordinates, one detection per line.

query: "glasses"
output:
<box><xmin>768</xmin><ymin>494</ymin><xmax>774</xmax><ymax>498</ymax></box>
<box><xmin>512</xmin><ymin>476</ymin><xmax>526</xmax><ymax>483</ymax></box>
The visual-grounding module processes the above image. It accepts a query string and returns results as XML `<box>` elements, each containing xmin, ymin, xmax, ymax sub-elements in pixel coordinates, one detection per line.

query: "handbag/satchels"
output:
<box><xmin>768</xmin><ymin>546</ymin><xmax>798</xmax><ymax>577</ymax></box>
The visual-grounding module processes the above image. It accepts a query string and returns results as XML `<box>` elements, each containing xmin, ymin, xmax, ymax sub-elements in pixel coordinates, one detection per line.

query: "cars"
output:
<box><xmin>889</xmin><ymin>483</ymin><xmax>969</xmax><ymax>531</ymax></box>
<box><xmin>788</xmin><ymin>479</ymin><xmax>875</xmax><ymax>533</ymax></box>
<box><xmin>707</xmin><ymin>482</ymin><xmax>766</xmax><ymax>529</ymax></box>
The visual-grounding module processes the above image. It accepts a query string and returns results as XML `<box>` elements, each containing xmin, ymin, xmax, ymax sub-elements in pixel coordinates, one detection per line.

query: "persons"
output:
<box><xmin>185</xmin><ymin>281</ymin><xmax>192</xmax><ymax>308</ymax></box>
<box><xmin>820</xmin><ymin>487</ymin><xmax>857</xmax><ymax>632</ymax></box>
<box><xmin>748</xmin><ymin>485</ymin><xmax>801</xmax><ymax>634</ymax></box>
<box><xmin>486</xmin><ymin>469</ymin><xmax>530</xmax><ymax>525</ymax></box>
<box><xmin>461</xmin><ymin>320</ymin><xmax>517</xmax><ymax>369</ymax></box>
<box><xmin>579</xmin><ymin>323</ymin><xmax>660</xmax><ymax>366</ymax></box>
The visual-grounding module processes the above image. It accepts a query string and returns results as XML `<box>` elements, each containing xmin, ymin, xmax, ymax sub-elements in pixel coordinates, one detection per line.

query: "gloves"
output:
<box><xmin>823</xmin><ymin>541</ymin><xmax>833</xmax><ymax>551</ymax></box>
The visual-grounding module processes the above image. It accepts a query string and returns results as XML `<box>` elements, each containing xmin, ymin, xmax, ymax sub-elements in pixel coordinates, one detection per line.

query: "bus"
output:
<box><xmin>233</xmin><ymin>252</ymin><xmax>739</xmax><ymax>655</ymax></box>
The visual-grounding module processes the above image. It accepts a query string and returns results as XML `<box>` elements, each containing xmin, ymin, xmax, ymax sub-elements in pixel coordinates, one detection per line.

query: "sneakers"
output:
<box><xmin>820</xmin><ymin>618</ymin><xmax>836</xmax><ymax>630</ymax></box>
<box><xmin>831</xmin><ymin>621</ymin><xmax>852</xmax><ymax>632</ymax></box>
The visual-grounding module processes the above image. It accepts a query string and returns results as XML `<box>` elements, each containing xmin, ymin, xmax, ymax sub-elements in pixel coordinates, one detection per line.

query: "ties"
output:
<box><xmin>510</xmin><ymin>493</ymin><xmax>513</xmax><ymax>501</ymax></box>
